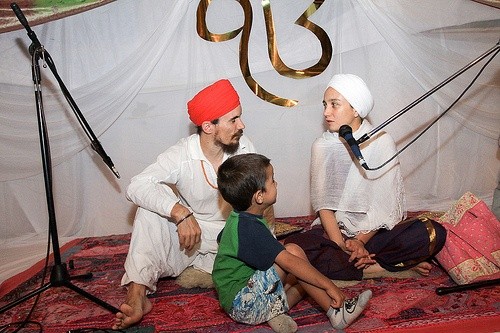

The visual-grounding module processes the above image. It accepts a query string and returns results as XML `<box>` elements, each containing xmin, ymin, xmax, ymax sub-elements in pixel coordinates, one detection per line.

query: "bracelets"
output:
<box><xmin>175</xmin><ymin>212</ymin><xmax>194</xmax><ymax>227</ymax></box>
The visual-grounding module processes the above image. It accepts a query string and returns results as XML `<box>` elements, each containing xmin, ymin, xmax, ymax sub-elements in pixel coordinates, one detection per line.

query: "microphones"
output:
<box><xmin>90</xmin><ymin>141</ymin><xmax>121</xmax><ymax>178</ymax></box>
<box><xmin>338</xmin><ymin>125</ymin><xmax>369</xmax><ymax>170</ymax></box>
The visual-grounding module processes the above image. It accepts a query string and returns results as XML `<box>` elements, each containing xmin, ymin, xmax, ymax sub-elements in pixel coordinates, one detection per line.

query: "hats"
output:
<box><xmin>326</xmin><ymin>74</ymin><xmax>374</xmax><ymax>118</ymax></box>
<box><xmin>187</xmin><ymin>79</ymin><xmax>241</xmax><ymax>126</ymax></box>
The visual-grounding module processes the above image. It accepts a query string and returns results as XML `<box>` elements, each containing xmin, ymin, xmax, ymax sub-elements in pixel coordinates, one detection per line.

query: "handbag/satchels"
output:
<box><xmin>366</xmin><ymin>215</ymin><xmax>446</xmax><ymax>271</ymax></box>
<box><xmin>433</xmin><ymin>191</ymin><xmax>500</xmax><ymax>286</ymax></box>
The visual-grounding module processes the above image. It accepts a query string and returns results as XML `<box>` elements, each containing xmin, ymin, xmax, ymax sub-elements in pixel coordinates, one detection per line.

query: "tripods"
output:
<box><xmin>1</xmin><ymin>4</ymin><xmax>120</xmax><ymax>315</ymax></box>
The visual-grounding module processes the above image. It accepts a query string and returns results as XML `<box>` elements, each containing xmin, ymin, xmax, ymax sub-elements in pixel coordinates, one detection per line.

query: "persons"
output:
<box><xmin>282</xmin><ymin>72</ymin><xmax>449</xmax><ymax>283</ymax></box>
<box><xmin>109</xmin><ymin>77</ymin><xmax>278</xmax><ymax>330</ymax></box>
<box><xmin>208</xmin><ymin>153</ymin><xmax>374</xmax><ymax>333</ymax></box>
